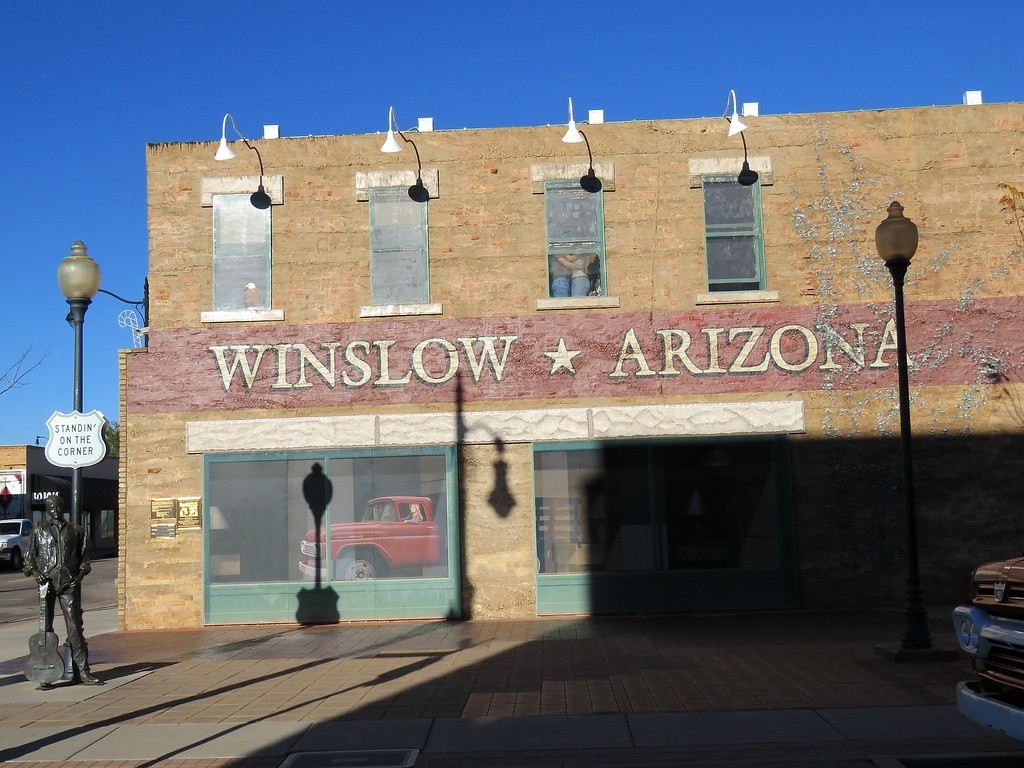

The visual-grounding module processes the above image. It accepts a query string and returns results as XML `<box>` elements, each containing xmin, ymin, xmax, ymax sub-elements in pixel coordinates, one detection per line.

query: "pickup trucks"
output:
<box><xmin>298</xmin><ymin>496</ymin><xmax>442</xmax><ymax>580</ymax></box>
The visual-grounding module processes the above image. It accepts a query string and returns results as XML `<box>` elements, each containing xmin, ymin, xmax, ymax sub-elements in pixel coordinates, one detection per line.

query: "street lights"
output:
<box><xmin>58</xmin><ymin>239</ymin><xmax>103</xmax><ymax>682</ymax></box>
<box><xmin>874</xmin><ymin>201</ymin><xmax>939</xmax><ymax>665</ymax></box>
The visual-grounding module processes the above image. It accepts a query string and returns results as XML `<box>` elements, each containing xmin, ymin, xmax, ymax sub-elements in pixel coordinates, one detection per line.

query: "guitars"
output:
<box><xmin>23</xmin><ymin>580</ymin><xmax>65</xmax><ymax>684</ymax></box>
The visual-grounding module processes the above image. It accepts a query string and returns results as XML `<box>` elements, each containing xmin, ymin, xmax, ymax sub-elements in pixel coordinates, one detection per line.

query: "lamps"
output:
<box><xmin>721</xmin><ymin>89</ymin><xmax>748</xmax><ymax>137</ymax></box>
<box><xmin>212</xmin><ymin>114</ymin><xmax>249</xmax><ymax>160</ymax></box>
<box><xmin>35</xmin><ymin>435</ymin><xmax>49</xmax><ymax>445</ymax></box>
<box><xmin>379</xmin><ymin>105</ymin><xmax>404</xmax><ymax>153</ymax></box>
<box><xmin>561</xmin><ymin>96</ymin><xmax>583</xmax><ymax>144</ymax></box>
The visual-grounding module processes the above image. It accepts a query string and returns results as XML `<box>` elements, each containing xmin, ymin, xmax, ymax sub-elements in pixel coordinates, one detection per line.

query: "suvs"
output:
<box><xmin>954</xmin><ymin>558</ymin><xmax>1024</xmax><ymax>742</ymax></box>
<box><xmin>0</xmin><ymin>518</ymin><xmax>35</xmax><ymax>572</ymax></box>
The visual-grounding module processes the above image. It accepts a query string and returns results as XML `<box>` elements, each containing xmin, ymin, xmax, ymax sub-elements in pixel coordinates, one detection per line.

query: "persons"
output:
<box><xmin>23</xmin><ymin>496</ymin><xmax>106</xmax><ymax>685</ymax></box>
